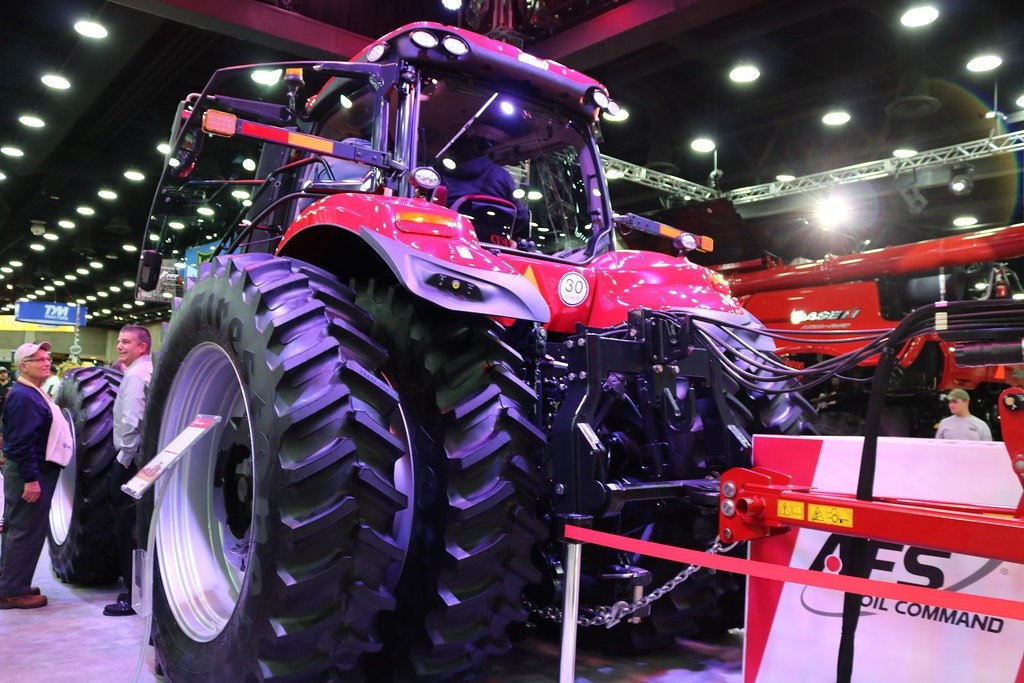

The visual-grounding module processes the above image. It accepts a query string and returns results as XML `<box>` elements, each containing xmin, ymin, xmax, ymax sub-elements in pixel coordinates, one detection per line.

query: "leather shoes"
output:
<box><xmin>103</xmin><ymin>593</ymin><xmax>137</xmax><ymax>616</ymax></box>
<box><xmin>0</xmin><ymin>587</ymin><xmax>46</xmax><ymax>609</ymax></box>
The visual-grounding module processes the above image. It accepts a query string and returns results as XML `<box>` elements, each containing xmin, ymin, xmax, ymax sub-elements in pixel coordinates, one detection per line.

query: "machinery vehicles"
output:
<box><xmin>47</xmin><ymin>21</ymin><xmax>818</xmax><ymax>683</ymax></box>
<box><xmin>704</xmin><ymin>222</ymin><xmax>1024</xmax><ymax>440</ymax></box>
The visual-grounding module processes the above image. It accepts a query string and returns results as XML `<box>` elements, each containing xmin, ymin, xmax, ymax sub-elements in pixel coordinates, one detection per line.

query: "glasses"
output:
<box><xmin>22</xmin><ymin>358</ymin><xmax>51</xmax><ymax>362</ymax></box>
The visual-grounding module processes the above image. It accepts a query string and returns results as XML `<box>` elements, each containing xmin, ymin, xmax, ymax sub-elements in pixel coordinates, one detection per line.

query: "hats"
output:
<box><xmin>14</xmin><ymin>341</ymin><xmax>51</xmax><ymax>364</ymax></box>
<box><xmin>0</xmin><ymin>366</ymin><xmax>8</xmax><ymax>372</ymax></box>
<box><xmin>944</xmin><ymin>388</ymin><xmax>970</xmax><ymax>401</ymax></box>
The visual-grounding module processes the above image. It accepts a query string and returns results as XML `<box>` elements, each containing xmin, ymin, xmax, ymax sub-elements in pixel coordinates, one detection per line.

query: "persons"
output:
<box><xmin>446</xmin><ymin>136</ymin><xmax>533</xmax><ymax>246</ymax></box>
<box><xmin>935</xmin><ymin>389</ymin><xmax>992</xmax><ymax>442</ymax></box>
<box><xmin>103</xmin><ymin>325</ymin><xmax>154</xmax><ymax>617</ymax></box>
<box><xmin>1</xmin><ymin>341</ymin><xmax>74</xmax><ymax>607</ymax></box>
<box><xmin>0</xmin><ymin>366</ymin><xmax>12</xmax><ymax>417</ymax></box>
